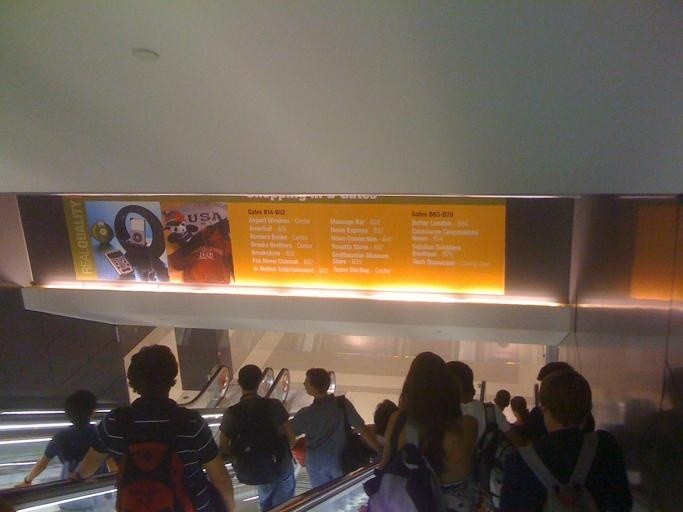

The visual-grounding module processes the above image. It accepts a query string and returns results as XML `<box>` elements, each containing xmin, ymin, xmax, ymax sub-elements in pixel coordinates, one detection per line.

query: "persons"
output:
<box><xmin>14</xmin><ymin>390</ymin><xmax>118</xmax><ymax>511</ymax></box>
<box><xmin>510</xmin><ymin>396</ymin><xmax>529</xmax><ymax>425</ymax></box>
<box><xmin>446</xmin><ymin>360</ymin><xmax>527</xmax><ymax>511</ymax></box>
<box><xmin>494</xmin><ymin>389</ymin><xmax>511</xmax><ymax>411</ymax></box>
<box><xmin>219</xmin><ymin>364</ymin><xmax>297</xmax><ymax>512</ymax></box>
<box><xmin>373</xmin><ymin>352</ymin><xmax>478</xmax><ymax>511</ymax></box>
<box><xmin>373</xmin><ymin>398</ymin><xmax>398</xmax><ymax>444</ymax></box>
<box><xmin>529</xmin><ymin>362</ymin><xmax>595</xmax><ymax>444</ymax></box>
<box><xmin>288</xmin><ymin>368</ymin><xmax>383</xmax><ymax>512</ymax></box>
<box><xmin>70</xmin><ymin>344</ymin><xmax>237</xmax><ymax>512</ymax></box>
<box><xmin>499</xmin><ymin>369</ymin><xmax>633</xmax><ymax>511</ymax></box>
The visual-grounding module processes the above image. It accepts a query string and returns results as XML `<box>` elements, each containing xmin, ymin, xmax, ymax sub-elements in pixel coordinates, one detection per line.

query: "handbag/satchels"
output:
<box><xmin>362</xmin><ymin>413</ymin><xmax>446</xmax><ymax>512</ymax></box>
<box><xmin>471</xmin><ymin>402</ymin><xmax>509</xmax><ymax>497</ymax></box>
<box><xmin>339</xmin><ymin>395</ymin><xmax>367</xmax><ymax>477</ymax></box>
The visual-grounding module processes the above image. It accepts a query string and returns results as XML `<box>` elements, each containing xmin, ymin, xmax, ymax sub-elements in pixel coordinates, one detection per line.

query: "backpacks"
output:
<box><xmin>112</xmin><ymin>407</ymin><xmax>191</xmax><ymax>511</ymax></box>
<box><xmin>520</xmin><ymin>433</ymin><xmax>605</xmax><ymax>511</ymax></box>
<box><xmin>222</xmin><ymin>399</ymin><xmax>284</xmax><ymax>485</ymax></box>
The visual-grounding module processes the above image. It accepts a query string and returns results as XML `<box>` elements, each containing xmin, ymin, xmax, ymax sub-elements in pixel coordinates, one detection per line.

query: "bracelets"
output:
<box><xmin>25</xmin><ymin>477</ymin><xmax>31</xmax><ymax>484</ymax></box>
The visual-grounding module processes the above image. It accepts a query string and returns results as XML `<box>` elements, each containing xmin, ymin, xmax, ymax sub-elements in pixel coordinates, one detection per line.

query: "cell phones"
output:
<box><xmin>105</xmin><ymin>249</ymin><xmax>133</xmax><ymax>276</ymax></box>
